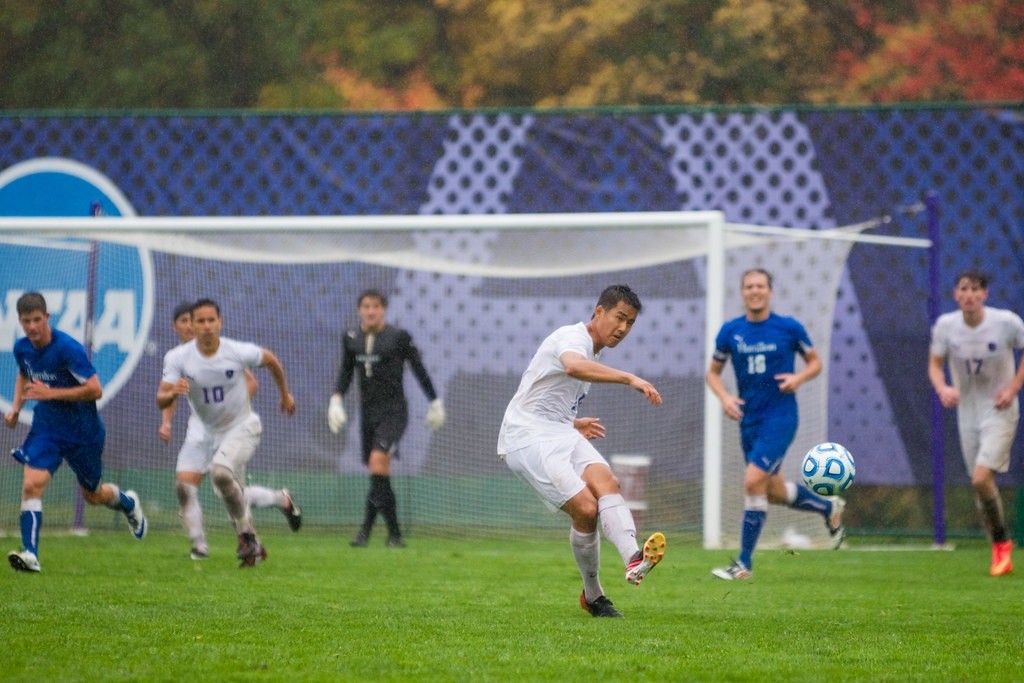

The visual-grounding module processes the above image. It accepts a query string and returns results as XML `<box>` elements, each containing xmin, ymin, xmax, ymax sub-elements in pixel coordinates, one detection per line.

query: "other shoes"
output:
<box><xmin>281</xmin><ymin>490</ymin><xmax>303</xmax><ymax>532</ymax></box>
<box><xmin>191</xmin><ymin>547</ymin><xmax>208</xmax><ymax>558</ymax></box>
<box><xmin>710</xmin><ymin>561</ymin><xmax>753</xmax><ymax>580</ymax></box>
<box><xmin>385</xmin><ymin>538</ymin><xmax>406</xmax><ymax>548</ymax></box>
<box><xmin>236</xmin><ymin>530</ymin><xmax>257</xmax><ymax>558</ymax></box>
<box><xmin>239</xmin><ymin>542</ymin><xmax>267</xmax><ymax>568</ymax></box>
<box><xmin>828</xmin><ymin>496</ymin><xmax>846</xmax><ymax>532</ymax></box>
<box><xmin>348</xmin><ymin>536</ymin><xmax>368</xmax><ymax>546</ymax></box>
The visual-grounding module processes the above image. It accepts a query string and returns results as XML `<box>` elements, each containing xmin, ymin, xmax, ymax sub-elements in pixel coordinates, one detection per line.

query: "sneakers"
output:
<box><xmin>580</xmin><ymin>589</ymin><xmax>623</xmax><ymax>617</ymax></box>
<box><xmin>125</xmin><ymin>490</ymin><xmax>147</xmax><ymax>540</ymax></box>
<box><xmin>7</xmin><ymin>549</ymin><xmax>41</xmax><ymax>572</ymax></box>
<box><xmin>626</xmin><ymin>532</ymin><xmax>667</xmax><ymax>586</ymax></box>
<box><xmin>990</xmin><ymin>538</ymin><xmax>1014</xmax><ymax>577</ymax></box>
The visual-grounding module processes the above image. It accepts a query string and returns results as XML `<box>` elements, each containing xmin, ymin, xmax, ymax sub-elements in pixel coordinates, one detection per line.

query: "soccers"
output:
<box><xmin>800</xmin><ymin>442</ymin><xmax>858</xmax><ymax>497</ymax></box>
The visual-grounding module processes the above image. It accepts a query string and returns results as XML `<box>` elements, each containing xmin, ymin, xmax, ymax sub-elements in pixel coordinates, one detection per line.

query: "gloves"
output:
<box><xmin>328</xmin><ymin>393</ymin><xmax>347</xmax><ymax>432</ymax></box>
<box><xmin>425</xmin><ymin>398</ymin><xmax>447</xmax><ymax>430</ymax></box>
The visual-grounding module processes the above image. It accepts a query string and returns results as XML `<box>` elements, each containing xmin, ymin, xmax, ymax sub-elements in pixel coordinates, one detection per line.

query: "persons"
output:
<box><xmin>5</xmin><ymin>291</ymin><xmax>148</xmax><ymax>573</ymax></box>
<box><xmin>928</xmin><ymin>271</ymin><xmax>1024</xmax><ymax>577</ymax></box>
<box><xmin>496</xmin><ymin>284</ymin><xmax>665</xmax><ymax>617</ymax></box>
<box><xmin>327</xmin><ymin>289</ymin><xmax>444</xmax><ymax>549</ymax></box>
<box><xmin>706</xmin><ymin>269</ymin><xmax>846</xmax><ymax>581</ymax></box>
<box><xmin>157</xmin><ymin>298</ymin><xmax>302</xmax><ymax>568</ymax></box>
